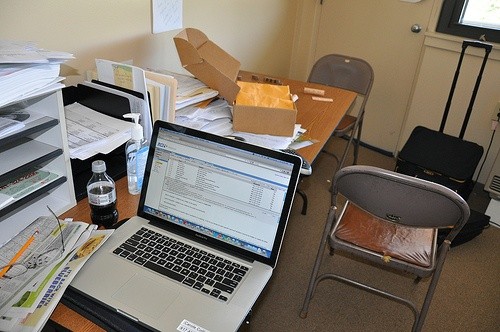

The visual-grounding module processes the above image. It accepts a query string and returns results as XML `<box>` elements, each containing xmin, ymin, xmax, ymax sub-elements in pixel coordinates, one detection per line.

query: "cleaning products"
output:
<box><xmin>123</xmin><ymin>111</ymin><xmax>150</xmax><ymax>195</ymax></box>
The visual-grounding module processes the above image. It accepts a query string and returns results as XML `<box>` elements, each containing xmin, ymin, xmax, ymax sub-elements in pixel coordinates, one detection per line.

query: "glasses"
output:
<box><xmin>0</xmin><ymin>205</ymin><xmax>65</xmax><ymax>279</ymax></box>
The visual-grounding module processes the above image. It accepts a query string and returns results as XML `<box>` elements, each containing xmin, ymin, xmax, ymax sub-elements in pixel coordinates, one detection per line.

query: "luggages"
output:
<box><xmin>393</xmin><ymin>40</ymin><xmax>492</xmax><ymax>205</ymax></box>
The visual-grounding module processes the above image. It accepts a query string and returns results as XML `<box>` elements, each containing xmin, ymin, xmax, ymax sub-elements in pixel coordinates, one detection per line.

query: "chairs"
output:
<box><xmin>308</xmin><ymin>53</ymin><xmax>375</xmax><ymax>170</ymax></box>
<box><xmin>299</xmin><ymin>166</ymin><xmax>472</xmax><ymax>331</ymax></box>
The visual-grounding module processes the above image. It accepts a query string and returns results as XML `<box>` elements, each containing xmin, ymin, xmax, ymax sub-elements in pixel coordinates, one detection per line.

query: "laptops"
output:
<box><xmin>62</xmin><ymin>120</ymin><xmax>302</xmax><ymax>332</ymax></box>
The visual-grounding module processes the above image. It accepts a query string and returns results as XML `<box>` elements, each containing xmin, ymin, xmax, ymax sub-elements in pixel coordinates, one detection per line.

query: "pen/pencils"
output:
<box><xmin>0</xmin><ymin>231</ymin><xmax>39</xmax><ymax>277</ymax></box>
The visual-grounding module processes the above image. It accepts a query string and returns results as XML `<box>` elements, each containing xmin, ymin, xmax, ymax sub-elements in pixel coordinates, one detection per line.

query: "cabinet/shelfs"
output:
<box><xmin>0</xmin><ymin>89</ymin><xmax>78</xmax><ymax>247</ymax></box>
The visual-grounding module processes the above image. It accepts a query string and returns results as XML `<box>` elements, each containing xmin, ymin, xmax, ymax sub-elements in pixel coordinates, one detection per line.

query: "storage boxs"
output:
<box><xmin>172</xmin><ymin>28</ymin><xmax>298</xmax><ymax>137</ymax></box>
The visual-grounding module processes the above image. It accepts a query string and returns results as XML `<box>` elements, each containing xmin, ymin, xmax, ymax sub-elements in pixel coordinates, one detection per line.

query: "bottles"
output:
<box><xmin>86</xmin><ymin>161</ymin><xmax>119</xmax><ymax>229</ymax></box>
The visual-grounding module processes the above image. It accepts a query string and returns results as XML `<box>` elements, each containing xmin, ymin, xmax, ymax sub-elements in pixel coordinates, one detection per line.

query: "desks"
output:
<box><xmin>40</xmin><ymin>69</ymin><xmax>358</xmax><ymax>332</ymax></box>
